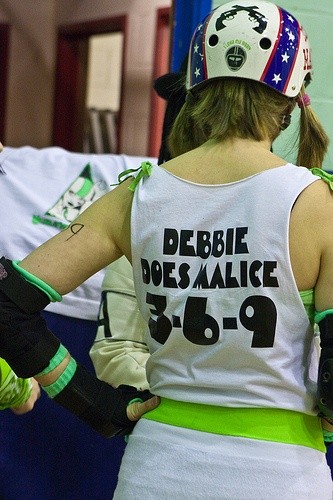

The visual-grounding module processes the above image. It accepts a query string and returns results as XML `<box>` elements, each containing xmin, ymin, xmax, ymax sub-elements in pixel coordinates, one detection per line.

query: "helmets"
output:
<box><xmin>183</xmin><ymin>0</ymin><xmax>314</xmax><ymax>98</ymax></box>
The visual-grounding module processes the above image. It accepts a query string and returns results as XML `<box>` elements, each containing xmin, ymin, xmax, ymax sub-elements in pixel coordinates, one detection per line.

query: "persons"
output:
<box><xmin>0</xmin><ymin>356</ymin><xmax>41</xmax><ymax>417</ymax></box>
<box><xmin>1</xmin><ymin>1</ymin><xmax>333</xmax><ymax>500</ymax></box>
